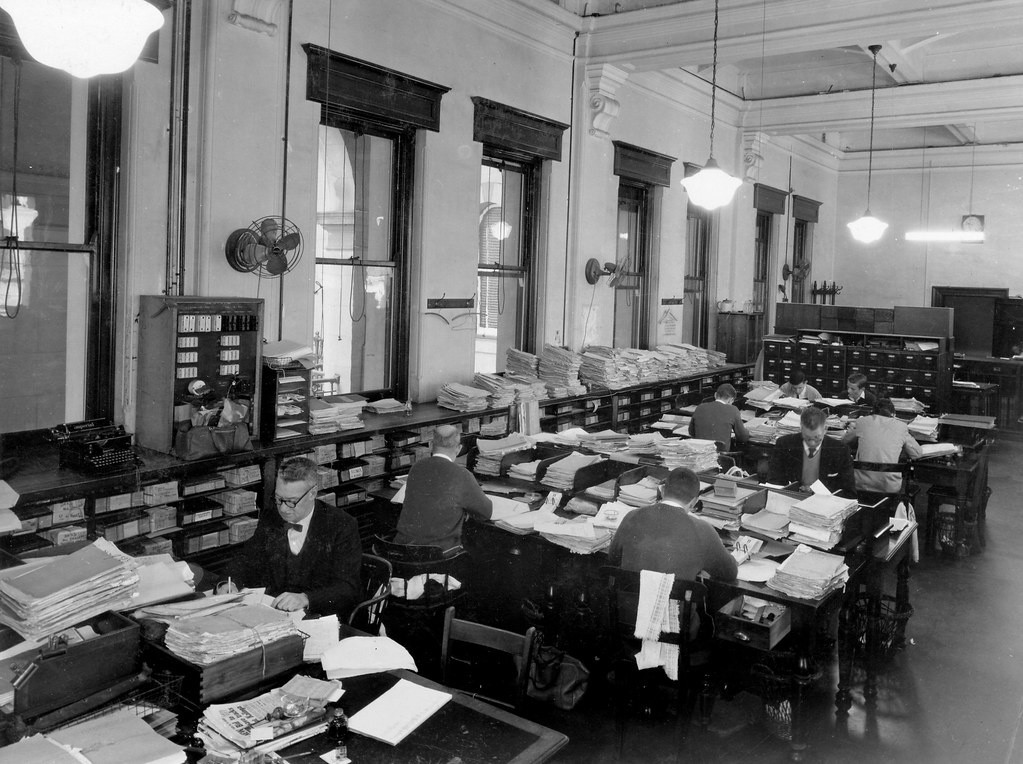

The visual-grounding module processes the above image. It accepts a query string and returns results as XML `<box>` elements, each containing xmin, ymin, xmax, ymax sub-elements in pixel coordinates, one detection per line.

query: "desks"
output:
<box><xmin>953</xmin><ymin>382</ymin><xmax>999</xmax><ymax>452</ymax></box>
<box><xmin>0</xmin><ymin>662</ymin><xmax>568</xmax><ymax>764</ymax></box>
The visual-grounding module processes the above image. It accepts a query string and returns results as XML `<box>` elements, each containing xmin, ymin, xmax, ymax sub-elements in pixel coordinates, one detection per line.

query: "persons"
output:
<box><xmin>768</xmin><ymin>407</ymin><xmax>858</xmax><ymax>499</ymax></box>
<box><xmin>214</xmin><ymin>456</ymin><xmax>367</xmax><ymax>626</ymax></box>
<box><xmin>610</xmin><ymin>468</ymin><xmax>735</xmax><ymax>702</ymax></box>
<box><xmin>688</xmin><ymin>385</ymin><xmax>749</xmax><ymax>450</ymax></box>
<box><xmin>839</xmin><ymin>374</ymin><xmax>876</xmax><ymax>405</ymax></box>
<box><xmin>841</xmin><ymin>400</ymin><xmax>922</xmax><ymax>492</ymax></box>
<box><xmin>781</xmin><ymin>371</ymin><xmax>822</xmax><ymax>401</ymax></box>
<box><xmin>394</xmin><ymin>425</ymin><xmax>494</xmax><ymax>619</ymax></box>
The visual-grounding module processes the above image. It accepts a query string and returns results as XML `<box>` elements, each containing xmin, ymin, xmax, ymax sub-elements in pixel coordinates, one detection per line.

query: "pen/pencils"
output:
<box><xmin>228</xmin><ymin>577</ymin><xmax>231</xmax><ymax>593</ymax></box>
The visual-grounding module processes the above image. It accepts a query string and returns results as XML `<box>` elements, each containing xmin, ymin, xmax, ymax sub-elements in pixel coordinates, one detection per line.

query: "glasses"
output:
<box><xmin>274</xmin><ymin>483</ymin><xmax>318</xmax><ymax>507</ymax></box>
<box><xmin>802</xmin><ymin>436</ymin><xmax>821</xmax><ymax>443</ymax></box>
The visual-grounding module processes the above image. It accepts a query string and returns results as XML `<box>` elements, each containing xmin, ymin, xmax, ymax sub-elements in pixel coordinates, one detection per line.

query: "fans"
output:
<box><xmin>225</xmin><ymin>215</ymin><xmax>305</xmax><ymax>279</ymax></box>
<box><xmin>782</xmin><ymin>257</ymin><xmax>812</xmax><ymax>283</ymax></box>
<box><xmin>585</xmin><ymin>256</ymin><xmax>632</xmax><ymax>289</ymax></box>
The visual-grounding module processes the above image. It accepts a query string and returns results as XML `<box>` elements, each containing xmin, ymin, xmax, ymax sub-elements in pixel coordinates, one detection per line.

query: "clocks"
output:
<box><xmin>961</xmin><ymin>215</ymin><xmax>985</xmax><ymax>244</ymax></box>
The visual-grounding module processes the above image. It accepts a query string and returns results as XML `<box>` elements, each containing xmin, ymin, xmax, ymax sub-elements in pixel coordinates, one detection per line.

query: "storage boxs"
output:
<box><xmin>775</xmin><ymin>303</ymin><xmax>954</xmax><ymax>337</ymax></box>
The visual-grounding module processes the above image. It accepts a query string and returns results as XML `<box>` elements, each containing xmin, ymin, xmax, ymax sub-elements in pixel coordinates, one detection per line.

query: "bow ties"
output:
<box><xmin>284</xmin><ymin>523</ymin><xmax>302</xmax><ymax>532</ymax></box>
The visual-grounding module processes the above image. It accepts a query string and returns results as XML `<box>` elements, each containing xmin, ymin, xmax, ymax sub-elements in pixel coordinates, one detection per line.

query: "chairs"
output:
<box><xmin>348</xmin><ymin>435</ymin><xmax>991</xmax><ymax>735</ymax></box>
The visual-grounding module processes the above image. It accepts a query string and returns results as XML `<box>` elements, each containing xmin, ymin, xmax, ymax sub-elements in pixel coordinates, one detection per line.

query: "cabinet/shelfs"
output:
<box><xmin>0</xmin><ymin>327</ymin><xmax>954</xmax><ymax>576</ymax></box>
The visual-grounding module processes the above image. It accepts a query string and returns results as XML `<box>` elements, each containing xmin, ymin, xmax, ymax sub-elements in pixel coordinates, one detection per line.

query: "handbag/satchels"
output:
<box><xmin>517</xmin><ymin>632</ymin><xmax>591</xmax><ymax>719</ymax></box>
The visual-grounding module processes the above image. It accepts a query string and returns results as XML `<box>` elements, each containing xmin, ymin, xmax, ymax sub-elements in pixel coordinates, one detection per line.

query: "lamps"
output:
<box><xmin>846</xmin><ymin>44</ymin><xmax>889</xmax><ymax>245</ymax></box>
<box><xmin>679</xmin><ymin>0</ymin><xmax>743</xmax><ymax>212</ymax></box>
<box><xmin>0</xmin><ymin>0</ymin><xmax>175</xmax><ymax>80</ymax></box>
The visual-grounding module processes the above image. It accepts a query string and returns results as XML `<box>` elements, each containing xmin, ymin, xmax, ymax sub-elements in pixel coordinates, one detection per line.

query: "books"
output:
<box><xmin>1</xmin><ymin>334</ymin><xmax>993</xmax><ymax>763</ymax></box>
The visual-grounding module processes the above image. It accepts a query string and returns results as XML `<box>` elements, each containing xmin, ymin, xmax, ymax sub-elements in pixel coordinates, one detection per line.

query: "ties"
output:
<box><xmin>797</xmin><ymin>395</ymin><xmax>799</xmax><ymax>398</ymax></box>
<box><xmin>809</xmin><ymin>448</ymin><xmax>816</xmax><ymax>458</ymax></box>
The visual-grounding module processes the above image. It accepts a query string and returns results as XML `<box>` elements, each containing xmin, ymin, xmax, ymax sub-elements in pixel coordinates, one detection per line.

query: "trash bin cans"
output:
<box><xmin>853</xmin><ymin>592</ymin><xmax>914</xmax><ymax>668</ymax></box>
<box><xmin>963</xmin><ymin>486</ymin><xmax>992</xmax><ymax>520</ymax></box>
<box><xmin>757</xmin><ymin>651</ymin><xmax>823</xmax><ymax>742</ymax></box>
<box><xmin>936</xmin><ymin>512</ymin><xmax>977</xmax><ymax>556</ymax></box>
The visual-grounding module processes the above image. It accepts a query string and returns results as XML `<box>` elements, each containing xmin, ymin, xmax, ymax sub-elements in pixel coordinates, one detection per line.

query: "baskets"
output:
<box><xmin>851</xmin><ymin>592</ymin><xmax>914</xmax><ymax>662</ymax></box>
<box><xmin>981</xmin><ymin>488</ymin><xmax>992</xmax><ymax>516</ymax></box>
<box><xmin>936</xmin><ymin>511</ymin><xmax>976</xmax><ymax>557</ymax></box>
